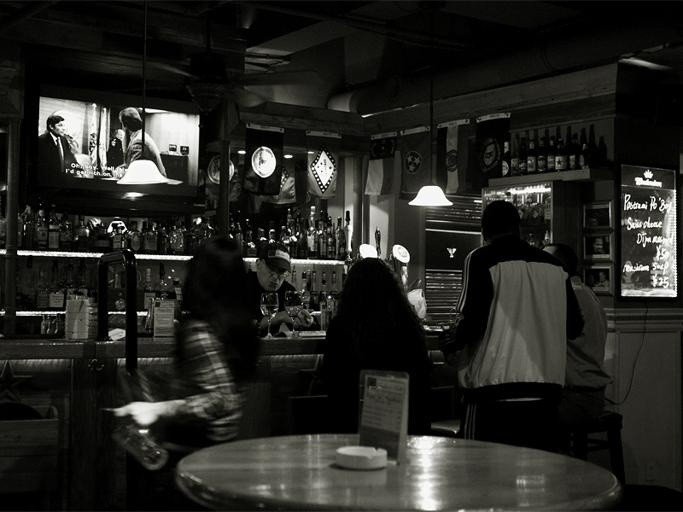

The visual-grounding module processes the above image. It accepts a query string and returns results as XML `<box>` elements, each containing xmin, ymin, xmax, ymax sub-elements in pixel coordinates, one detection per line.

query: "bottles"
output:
<box><xmin>34</xmin><ymin>272</ymin><xmax>88</xmax><ymax>310</ymax></box>
<box><xmin>287</xmin><ymin>269</ymin><xmax>338</xmax><ymax>331</ymax></box>
<box><xmin>19</xmin><ymin>202</ymin><xmax>347</xmax><ymax>262</ymax></box>
<box><xmin>500</xmin><ymin>124</ymin><xmax>599</xmax><ymax>176</ymax></box>
<box><xmin>40</xmin><ymin>313</ymin><xmax>62</xmax><ymax>336</ymax></box>
<box><xmin>141</xmin><ymin>268</ymin><xmax>179</xmax><ymax>310</ymax></box>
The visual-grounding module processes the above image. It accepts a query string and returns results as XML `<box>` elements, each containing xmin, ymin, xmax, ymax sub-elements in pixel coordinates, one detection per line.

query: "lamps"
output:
<box><xmin>407</xmin><ymin>0</ymin><xmax>454</xmax><ymax>211</ymax></box>
<box><xmin>108</xmin><ymin>1</ymin><xmax>183</xmax><ymax>186</ymax></box>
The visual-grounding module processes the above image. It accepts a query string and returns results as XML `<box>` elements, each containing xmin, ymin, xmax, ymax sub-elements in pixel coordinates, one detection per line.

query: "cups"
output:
<box><xmin>112</xmin><ymin>423</ymin><xmax>168</xmax><ymax>471</ymax></box>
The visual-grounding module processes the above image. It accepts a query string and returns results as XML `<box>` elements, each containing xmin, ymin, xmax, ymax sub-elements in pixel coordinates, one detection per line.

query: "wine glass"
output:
<box><xmin>260</xmin><ymin>292</ymin><xmax>279</xmax><ymax>339</ymax></box>
<box><xmin>283</xmin><ymin>291</ymin><xmax>302</xmax><ymax>338</ymax></box>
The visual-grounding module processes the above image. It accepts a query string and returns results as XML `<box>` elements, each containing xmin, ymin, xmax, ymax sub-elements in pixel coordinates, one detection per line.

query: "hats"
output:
<box><xmin>262</xmin><ymin>242</ymin><xmax>291</xmax><ymax>271</ymax></box>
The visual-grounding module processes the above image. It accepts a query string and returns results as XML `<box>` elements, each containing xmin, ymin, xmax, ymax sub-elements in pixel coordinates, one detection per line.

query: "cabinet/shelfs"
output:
<box><xmin>1</xmin><ymin>243</ymin><xmax>346</xmax><ymax>320</ymax></box>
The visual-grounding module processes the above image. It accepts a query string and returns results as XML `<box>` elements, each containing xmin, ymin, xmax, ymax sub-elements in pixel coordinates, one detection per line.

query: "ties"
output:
<box><xmin>56</xmin><ymin>137</ymin><xmax>65</xmax><ymax>173</ymax></box>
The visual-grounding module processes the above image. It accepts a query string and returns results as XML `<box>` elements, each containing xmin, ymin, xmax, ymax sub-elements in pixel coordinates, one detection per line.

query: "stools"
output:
<box><xmin>571</xmin><ymin>405</ymin><xmax>628</xmax><ymax>488</ymax></box>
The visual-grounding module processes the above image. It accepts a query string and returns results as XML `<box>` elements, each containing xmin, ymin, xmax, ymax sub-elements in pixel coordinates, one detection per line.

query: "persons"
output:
<box><xmin>114</xmin><ymin>237</ymin><xmax>250</xmax><ymax>447</ymax></box>
<box><xmin>541</xmin><ymin>243</ymin><xmax>609</xmax><ymax>409</ymax></box>
<box><xmin>40</xmin><ymin>114</ymin><xmax>76</xmax><ymax>175</ymax></box>
<box><xmin>115</xmin><ymin>108</ymin><xmax>167</xmax><ymax>181</ymax></box>
<box><xmin>249</xmin><ymin>240</ymin><xmax>321</xmax><ymax>332</ymax></box>
<box><xmin>443</xmin><ymin>198</ymin><xmax>585</xmax><ymax>451</ymax></box>
<box><xmin>319</xmin><ymin>259</ymin><xmax>432</xmax><ymax>436</ymax></box>
<box><xmin>107</xmin><ymin>127</ymin><xmax>126</xmax><ymax>167</ymax></box>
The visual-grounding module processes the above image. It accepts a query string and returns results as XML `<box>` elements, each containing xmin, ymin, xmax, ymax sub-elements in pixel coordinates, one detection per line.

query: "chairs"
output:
<box><xmin>463</xmin><ymin>381</ymin><xmax>568</xmax><ymax>454</ymax></box>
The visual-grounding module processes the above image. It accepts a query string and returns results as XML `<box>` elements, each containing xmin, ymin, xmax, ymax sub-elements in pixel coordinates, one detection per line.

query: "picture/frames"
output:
<box><xmin>580</xmin><ymin>203</ymin><xmax>617</xmax><ymax>298</ymax></box>
